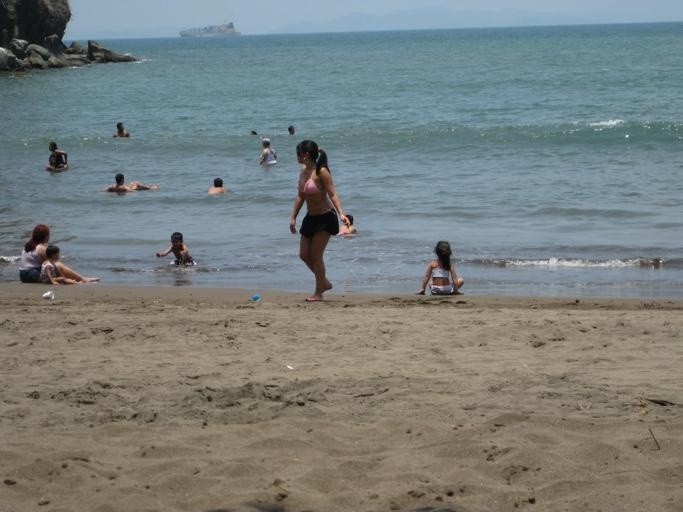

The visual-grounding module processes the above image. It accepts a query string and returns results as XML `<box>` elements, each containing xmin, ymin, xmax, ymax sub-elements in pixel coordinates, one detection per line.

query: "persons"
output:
<box><xmin>249</xmin><ymin>131</ymin><xmax>258</xmax><ymax>136</ymax></box>
<box><xmin>113</xmin><ymin>122</ymin><xmax>129</xmax><ymax>137</ymax></box>
<box><xmin>40</xmin><ymin>245</ymin><xmax>81</xmax><ymax>285</ymax></box>
<box><xmin>17</xmin><ymin>224</ymin><xmax>102</xmax><ymax>285</ymax></box>
<box><xmin>154</xmin><ymin>231</ymin><xmax>195</xmax><ymax>266</ymax></box>
<box><xmin>288</xmin><ymin>124</ymin><xmax>296</xmax><ymax>136</ymax></box>
<box><xmin>260</xmin><ymin>137</ymin><xmax>276</xmax><ymax>167</ymax></box>
<box><xmin>288</xmin><ymin>139</ymin><xmax>350</xmax><ymax>302</ymax></box>
<box><xmin>415</xmin><ymin>240</ymin><xmax>464</xmax><ymax>295</ymax></box>
<box><xmin>206</xmin><ymin>178</ymin><xmax>227</xmax><ymax>195</ymax></box>
<box><xmin>102</xmin><ymin>173</ymin><xmax>159</xmax><ymax>193</ymax></box>
<box><xmin>336</xmin><ymin>215</ymin><xmax>358</xmax><ymax>235</ymax></box>
<box><xmin>45</xmin><ymin>141</ymin><xmax>68</xmax><ymax>172</ymax></box>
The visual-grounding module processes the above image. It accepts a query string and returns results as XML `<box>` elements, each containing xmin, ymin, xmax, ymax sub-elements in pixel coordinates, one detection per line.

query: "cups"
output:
<box><xmin>41</xmin><ymin>291</ymin><xmax>53</xmax><ymax>298</ymax></box>
